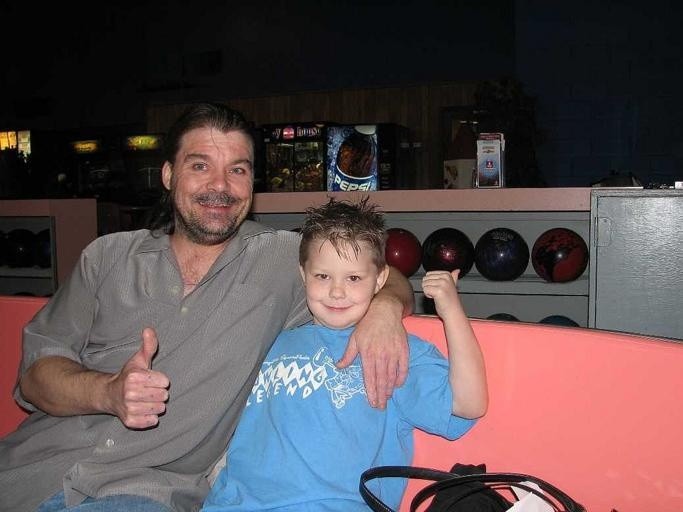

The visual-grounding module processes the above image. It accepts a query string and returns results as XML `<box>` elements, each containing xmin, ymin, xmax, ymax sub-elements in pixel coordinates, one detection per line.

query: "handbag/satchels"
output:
<box><xmin>359</xmin><ymin>465</ymin><xmax>586</xmax><ymax>512</ymax></box>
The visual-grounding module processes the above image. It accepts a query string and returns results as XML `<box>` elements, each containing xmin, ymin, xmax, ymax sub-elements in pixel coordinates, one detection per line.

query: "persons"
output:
<box><xmin>0</xmin><ymin>98</ymin><xmax>418</xmax><ymax>512</ymax></box>
<box><xmin>201</xmin><ymin>191</ymin><xmax>490</xmax><ymax>512</ymax></box>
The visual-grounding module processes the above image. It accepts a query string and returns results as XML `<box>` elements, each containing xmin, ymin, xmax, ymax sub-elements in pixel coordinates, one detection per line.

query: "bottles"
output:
<box><xmin>332</xmin><ymin>125</ymin><xmax>376</xmax><ymax>191</ymax></box>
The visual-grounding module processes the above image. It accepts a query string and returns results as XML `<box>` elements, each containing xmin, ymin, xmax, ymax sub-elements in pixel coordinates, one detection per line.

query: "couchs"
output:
<box><xmin>0</xmin><ymin>296</ymin><xmax>683</xmax><ymax>512</ymax></box>
